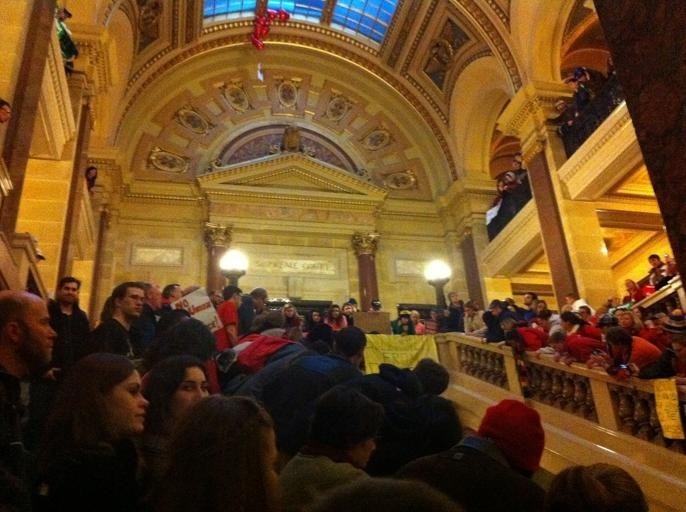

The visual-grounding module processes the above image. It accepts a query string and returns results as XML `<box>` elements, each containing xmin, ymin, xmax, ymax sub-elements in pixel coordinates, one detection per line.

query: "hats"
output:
<box><xmin>309</xmin><ymin>384</ymin><xmax>382</xmax><ymax>444</ymax></box>
<box><xmin>661</xmin><ymin>309</ymin><xmax>686</xmax><ymax>333</ymax></box>
<box><xmin>573</xmin><ymin>68</ymin><xmax>585</xmax><ymax>79</ymax></box>
<box><xmin>474</xmin><ymin>398</ymin><xmax>546</xmax><ymax>471</ymax></box>
<box><xmin>414</xmin><ymin>357</ymin><xmax>450</xmax><ymax>396</ymax></box>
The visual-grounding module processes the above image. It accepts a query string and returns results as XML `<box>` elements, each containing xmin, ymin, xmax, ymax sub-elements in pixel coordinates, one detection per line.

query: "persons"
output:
<box><xmin>144</xmin><ymin>320</ymin><xmax>214</xmax><ymax>371</ymax></box>
<box><xmin>221</xmin><ymin>323</ymin><xmax>334</xmax><ymax>400</ymax></box>
<box><xmin>351</xmin><ymin>358</ymin><xmax>449</xmax><ymax>419</ymax></box>
<box><xmin>379</xmin><ymin>395</ymin><xmax>464</xmax><ymax>478</ymax></box>
<box><xmin>31</xmin><ymin>277</ymin><xmax>89</xmax><ymax>445</ymax></box>
<box><xmin>142</xmin><ymin>393</ymin><xmax>271</xmax><ymax>511</ymax></box>
<box><xmin>86</xmin><ymin>166</ymin><xmax>97</xmax><ymax>189</ymax></box>
<box><xmin>396</xmin><ymin>398</ymin><xmax>549</xmax><ymax>511</ymax></box>
<box><xmin>341</xmin><ymin>302</ymin><xmax>354</xmax><ymax>324</ymax></box>
<box><xmin>542</xmin><ymin>59</ymin><xmax>624</xmax><ymax>162</ymax></box>
<box><xmin>325</xmin><ymin>304</ymin><xmax>347</xmax><ymax>328</ymax></box>
<box><xmin>261</xmin><ymin>326</ymin><xmax>366</xmax><ymax>428</ymax></box>
<box><xmin>26</xmin><ymin>354</ymin><xmax>148</xmax><ymax>511</ymax></box>
<box><xmin>284</xmin><ymin>303</ymin><xmax>301</xmax><ymax>327</ymax></box>
<box><xmin>0</xmin><ymin>97</ymin><xmax>11</xmax><ymax>122</ymax></box>
<box><xmin>58</xmin><ymin>6</ymin><xmax>80</xmax><ymax>74</ymax></box>
<box><xmin>307</xmin><ymin>310</ymin><xmax>328</xmax><ymax>327</ymax></box>
<box><xmin>549</xmin><ymin>463</ymin><xmax>648</xmax><ymax>512</ymax></box>
<box><xmin>273</xmin><ymin>382</ymin><xmax>380</xmax><ymax>512</ymax></box>
<box><xmin>394</xmin><ymin>310</ymin><xmax>415</xmax><ymax>335</ymax></box>
<box><xmin>214</xmin><ymin>286</ymin><xmax>242</xmax><ymax>353</ymax></box>
<box><xmin>162</xmin><ymin>283</ymin><xmax>183</xmax><ymax>306</ymax></box>
<box><xmin>146</xmin><ymin>356</ymin><xmax>210</xmax><ymax>490</ymax></box>
<box><xmin>549</xmin><ymin>254</ymin><xmax>686</xmax><ymax>385</ymax></box>
<box><xmin>81</xmin><ymin>282</ymin><xmax>145</xmax><ymax>358</ymax></box>
<box><xmin>410</xmin><ymin>310</ymin><xmax>425</xmax><ymax>334</ymax></box>
<box><xmin>426</xmin><ymin>291</ymin><xmax>548</xmax><ymax>350</ymax></box>
<box><xmin>238</xmin><ymin>288</ymin><xmax>267</xmax><ymax>337</ymax></box>
<box><xmin>131</xmin><ymin>282</ymin><xmax>162</xmax><ymax>353</ymax></box>
<box><xmin>222</xmin><ymin>327</ymin><xmax>301</xmax><ymax>387</ymax></box>
<box><xmin>490</xmin><ymin>152</ymin><xmax>531</xmax><ymax>212</ymax></box>
<box><xmin>0</xmin><ymin>288</ymin><xmax>58</xmax><ymax>511</ymax></box>
